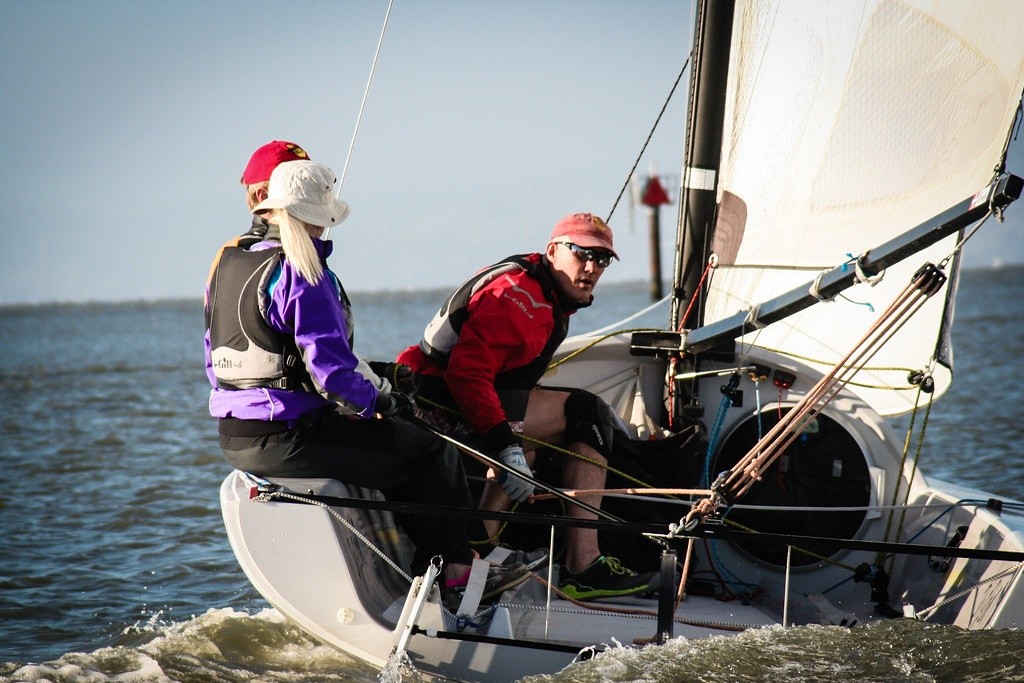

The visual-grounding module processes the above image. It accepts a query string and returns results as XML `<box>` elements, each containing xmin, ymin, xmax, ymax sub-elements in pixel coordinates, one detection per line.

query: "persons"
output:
<box><xmin>397</xmin><ymin>214</ymin><xmax>660</xmax><ymax>600</ymax></box>
<box><xmin>204</xmin><ymin>141</ymin><xmax>549</xmax><ymax>612</ymax></box>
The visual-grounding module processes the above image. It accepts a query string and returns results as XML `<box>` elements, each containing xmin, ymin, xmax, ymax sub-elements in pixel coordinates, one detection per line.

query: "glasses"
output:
<box><xmin>556</xmin><ymin>242</ymin><xmax>614</xmax><ymax>268</ymax></box>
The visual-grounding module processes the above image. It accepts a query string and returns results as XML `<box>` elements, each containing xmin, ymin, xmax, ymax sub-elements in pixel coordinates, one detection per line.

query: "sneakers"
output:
<box><xmin>466</xmin><ymin>541</ymin><xmax>551</xmax><ymax>572</ymax></box>
<box><xmin>557</xmin><ymin>554</ymin><xmax>661</xmax><ymax>600</ymax></box>
<box><xmin>455</xmin><ymin>560</ymin><xmax>531</xmax><ymax>600</ymax></box>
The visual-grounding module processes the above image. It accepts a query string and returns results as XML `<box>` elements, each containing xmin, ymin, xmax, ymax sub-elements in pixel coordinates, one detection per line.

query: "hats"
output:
<box><xmin>549</xmin><ymin>212</ymin><xmax>620</xmax><ymax>262</ymax></box>
<box><xmin>251</xmin><ymin>159</ymin><xmax>351</xmax><ymax>227</ymax></box>
<box><xmin>240</xmin><ymin>140</ymin><xmax>311</xmax><ymax>185</ymax></box>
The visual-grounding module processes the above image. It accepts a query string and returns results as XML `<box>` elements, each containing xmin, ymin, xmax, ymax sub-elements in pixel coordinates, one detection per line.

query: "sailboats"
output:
<box><xmin>220</xmin><ymin>0</ymin><xmax>1024</xmax><ymax>679</ymax></box>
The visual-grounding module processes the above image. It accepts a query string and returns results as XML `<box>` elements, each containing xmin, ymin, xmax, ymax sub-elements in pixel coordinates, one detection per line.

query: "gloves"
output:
<box><xmin>498</xmin><ymin>447</ymin><xmax>535</xmax><ymax>503</ymax></box>
<box><xmin>370</xmin><ymin>357</ymin><xmax>420</xmax><ymax>421</ymax></box>
